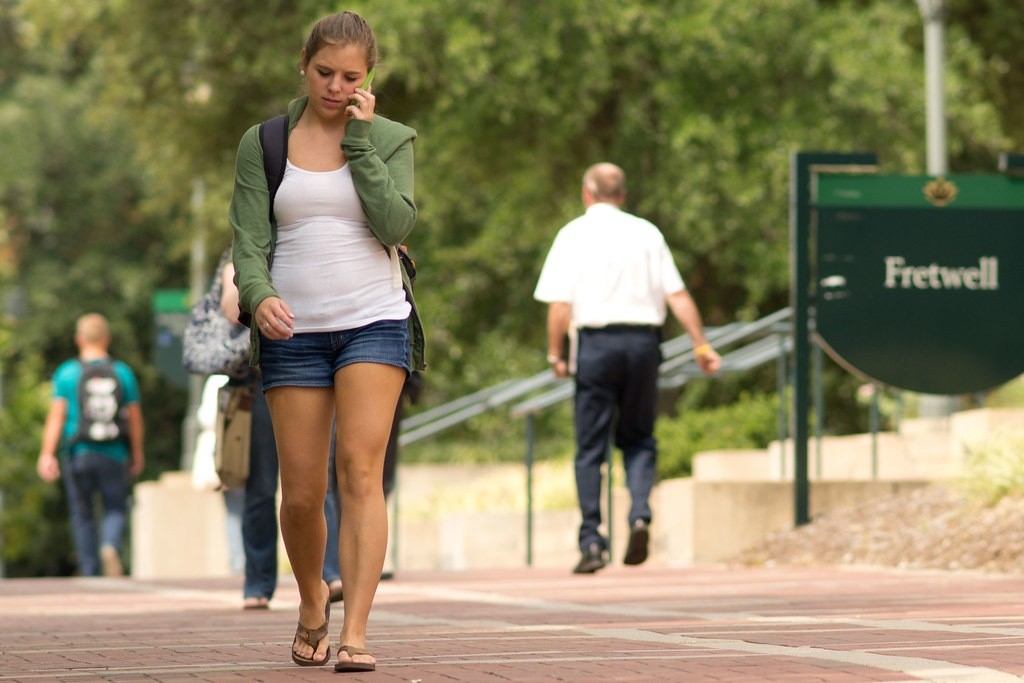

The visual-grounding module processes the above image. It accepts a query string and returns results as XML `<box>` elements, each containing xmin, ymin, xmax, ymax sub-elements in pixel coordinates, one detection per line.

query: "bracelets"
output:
<box><xmin>546</xmin><ymin>354</ymin><xmax>565</xmax><ymax>368</ymax></box>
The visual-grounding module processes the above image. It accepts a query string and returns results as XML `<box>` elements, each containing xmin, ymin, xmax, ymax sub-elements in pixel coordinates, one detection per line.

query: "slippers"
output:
<box><xmin>245</xmin><ymin>596</ymin><xmax>267</xmax><ymax>609</ymax></box>
<box><xmin>292</xmin><ymin>599</ymin><xmax>331</xmax><ymax>666</ymax></box>
<box><xmin>330</xmin><ymin>572</ymin><xmax>391</xmax><ymax>603</ymax></box>
<box><xmin>334</xmin><ymin>645</ymin><xmax>375</xmax><ymax>671</ymax></box>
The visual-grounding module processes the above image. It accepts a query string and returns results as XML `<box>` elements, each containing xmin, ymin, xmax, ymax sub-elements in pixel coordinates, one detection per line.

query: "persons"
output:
<box><xmin>228</xmin><ymin>11</ymin><xmax>426</xmax><ymax>671</ymax></box>
<box><xmin>205</xmin><ymin>247</ymin><xmax>347</xmax><ymax>609</ymax></box>
<box><xmin>37</xmin><ymin>311</ymin><xmax>145</xmax><ymax>580</ymax></box>
<box><xmin>531</xmin><ymin>161</ymin><xmax>726</xmax><ymax>575</ymax></box>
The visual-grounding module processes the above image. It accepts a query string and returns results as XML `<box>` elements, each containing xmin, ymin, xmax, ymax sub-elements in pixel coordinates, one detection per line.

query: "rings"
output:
<box><xmin>265</xmin><ymin>323</ymin><xmax>269</xmax><ymax>329</ymax></box>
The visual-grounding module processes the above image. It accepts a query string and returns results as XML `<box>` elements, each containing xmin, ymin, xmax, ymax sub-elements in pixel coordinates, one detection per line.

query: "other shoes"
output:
<box><xmin>624</xmin><ymin>519</ymin><xmax>649</xmax><ymax>565</ymax></box>
<box><xmin>101</xmin><ymin>544</ymin><xmax>125</xmax><ymax>576</ymax></box>
<box><xmin>575</xmin><ymin>543</ymin><xmax>604</xmax><ymax>571</ymax></box>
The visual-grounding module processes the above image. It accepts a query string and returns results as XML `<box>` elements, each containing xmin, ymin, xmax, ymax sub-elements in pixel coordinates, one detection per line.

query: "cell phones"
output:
<box><xmin>350</xmin><ymin>68</ymin><xmax>376</xmax><ymax>108</ymax></box>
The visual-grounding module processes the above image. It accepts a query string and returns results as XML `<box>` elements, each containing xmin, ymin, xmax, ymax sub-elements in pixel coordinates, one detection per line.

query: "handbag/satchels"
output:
<box><xmin>191</xmin><ymin>374</ymin><xmax>229</xmax><ymax>491</ymax></box>
<box><xmin>210</xmin><ymin>355</ymin><xmax>261</xmax><ymax>493</ymax></box>
<box><xmin>181</xmin><ymin>251</ymin><xmax>252</xmax><ymax>375</ymax></box>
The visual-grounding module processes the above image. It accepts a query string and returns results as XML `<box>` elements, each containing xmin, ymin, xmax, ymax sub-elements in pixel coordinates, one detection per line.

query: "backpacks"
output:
<box><xmin>67</xmin><ymin>357</ymin><xmax>132</xmax><ymax>453</ymax></box>
